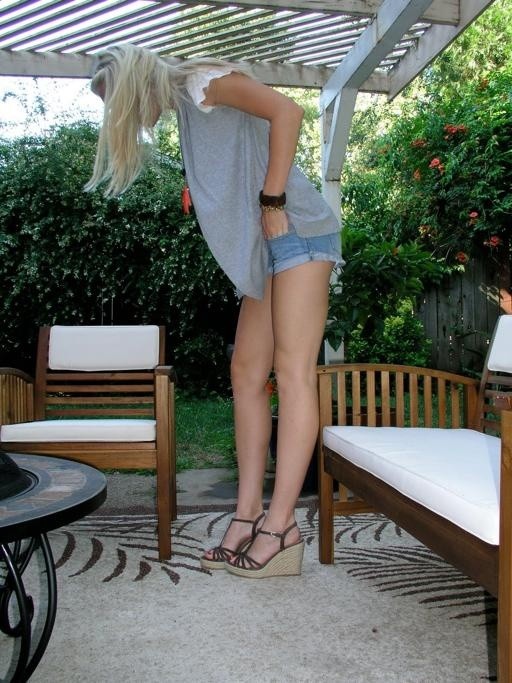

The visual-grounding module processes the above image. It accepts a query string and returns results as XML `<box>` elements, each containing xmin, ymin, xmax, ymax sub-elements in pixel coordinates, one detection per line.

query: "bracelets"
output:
<box><xmin>259</xmin><ymin>189</ymin><xmax>286</xmax><ymax>208</ymax></box>
<box><xmin>260</xmin><ymin>204</ymin><xmax>285</xmax><ymax>213</ymax></box>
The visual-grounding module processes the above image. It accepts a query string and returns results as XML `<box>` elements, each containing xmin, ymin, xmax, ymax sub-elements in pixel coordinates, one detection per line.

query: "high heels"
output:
<box><xmin>200</xmin><ymin>512</ymin><xmax>305</xmax><ymax>579</ymax></box>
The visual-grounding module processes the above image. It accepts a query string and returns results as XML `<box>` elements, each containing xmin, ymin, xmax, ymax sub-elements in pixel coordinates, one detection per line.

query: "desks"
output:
<box><xmin>0</xmin><ymin>451</ymin><xmax>108</xmax><ymax>683</ymax></box>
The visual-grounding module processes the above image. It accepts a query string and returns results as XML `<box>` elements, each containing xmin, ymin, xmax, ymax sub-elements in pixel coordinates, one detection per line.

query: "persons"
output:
<box><xmin>79</xmin><ymin>43</ymin><xmax>348</xmax><ymax>578</ymax></box>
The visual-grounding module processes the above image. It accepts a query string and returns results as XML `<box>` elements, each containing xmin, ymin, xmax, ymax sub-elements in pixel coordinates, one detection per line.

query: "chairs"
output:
<box><xmin>0</xmin><ymin>323</ymin><xmax>176</xmax><ymax>558</ymax></box>
<box><xmin>314</xmin><ymin>314</ymin><xmax>512</xmax><ymax>682</ymax></box>
